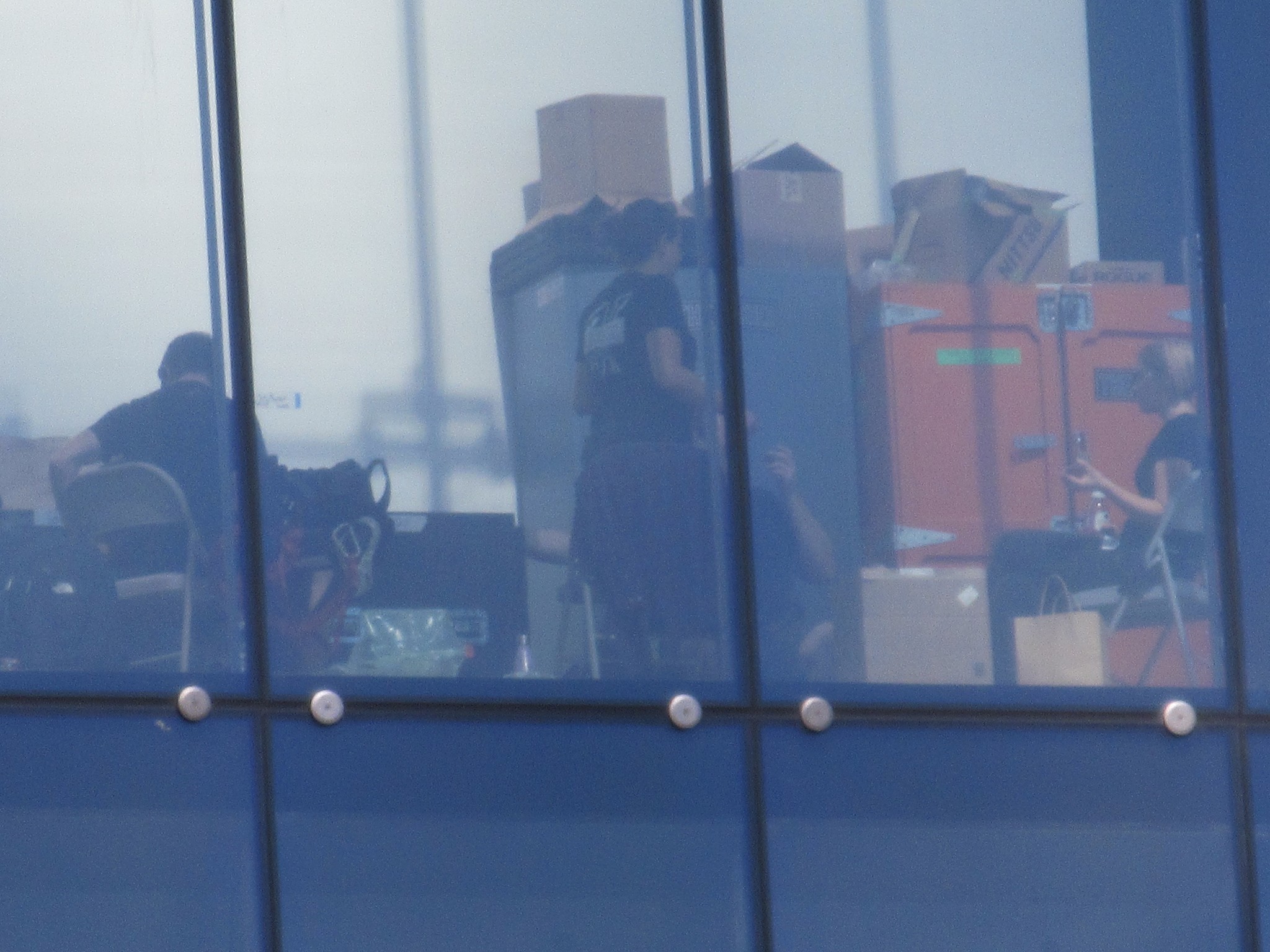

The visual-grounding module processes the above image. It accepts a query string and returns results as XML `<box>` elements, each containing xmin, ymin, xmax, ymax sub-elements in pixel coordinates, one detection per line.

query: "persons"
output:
<box><xmin>984</xmin><ymin>338</ymin><xmax>1204</xmax><ymax>691</ymax></box>
<box><xmin>576</xmin><ymin>197</ymin><xmax>842</xmax><ymax>685</ymax></box>
<box><xmin>47</xmin><ymin>332</ymin><xmax>277</xmax><ymax>593</ymax></box>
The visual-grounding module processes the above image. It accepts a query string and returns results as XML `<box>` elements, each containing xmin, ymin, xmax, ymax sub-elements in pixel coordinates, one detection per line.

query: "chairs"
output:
<box><xmin>1068</xmin><ymin>470</ymin><xmax>1207</xmax><ymax>683</ymax></box>
<box><xmin>64</xmin><ymin>462</ymin><xmax>230</xmax><ymax>674</ymax></box>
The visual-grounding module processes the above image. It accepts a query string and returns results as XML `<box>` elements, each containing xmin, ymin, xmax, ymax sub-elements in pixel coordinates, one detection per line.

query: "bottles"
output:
<box><xmin>1088</xmin><ymin>489</ymin><xmax>1114</xmax><ymax>551</ymax></box>
